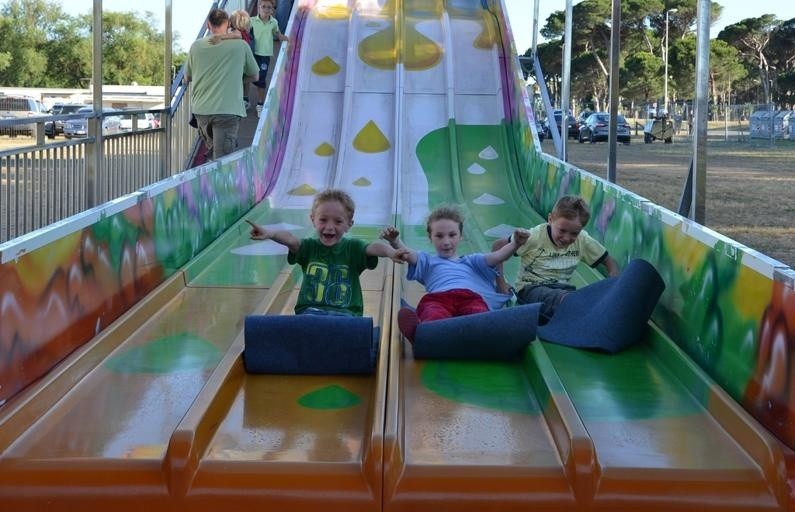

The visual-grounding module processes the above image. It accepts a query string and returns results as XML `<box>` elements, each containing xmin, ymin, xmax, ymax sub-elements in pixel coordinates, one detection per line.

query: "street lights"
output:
<box><xmin>664</xmin><ymin>8</ymin><xmax>679</xmax><ymax>113</ymax></box>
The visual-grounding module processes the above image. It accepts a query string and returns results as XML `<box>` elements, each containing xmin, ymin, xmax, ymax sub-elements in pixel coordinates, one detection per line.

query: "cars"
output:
<box><xmin>0</xmin><ymin>92</ymin><xmax>166</xmax><ymax>140</ymax></box>
<box><xmin>535</xmin><ymin>108</ymin><xmax>631</xmax><ymax>145</ymax></box>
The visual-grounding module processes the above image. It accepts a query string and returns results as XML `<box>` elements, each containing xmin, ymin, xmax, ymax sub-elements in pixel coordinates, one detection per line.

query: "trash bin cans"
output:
<box><xmin>749</xmin><ymin>111</ymin><xmax>795</xmax><ymax>139</ymax></box>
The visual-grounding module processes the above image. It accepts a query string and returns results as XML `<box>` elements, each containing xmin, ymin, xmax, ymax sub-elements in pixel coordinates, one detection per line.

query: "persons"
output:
<box><xmin>183</xmin><ymin>9</ymin><xmax>260</xmax><ymax>165</ymax></box>
<box><xmin>491</xmin><ymin>194</ymin><xmax>621</xmax><ymax>326</ymax></box>
<box><xmin>242</xmin><ymin>1</ymin><xmax>289</xmax><ymax>120</ymax></box>
<box><xmin>271</xmin><ymin>0</ymin><xmax>294</xmax><ymax>43</ymax></box>
<box><xmin>207</xmin><ymin>10</ymin><xmax>252</xmax><ymax>46</ymax></box>
<box><xmin>376</xmin><ymin>205</ymin><xmax>530</xmax><ymax>345</ymax></box>
<box><xmin>244</xmin><ymin>189</ymin><xmax>412</xmax><ymax>317</ymax></box>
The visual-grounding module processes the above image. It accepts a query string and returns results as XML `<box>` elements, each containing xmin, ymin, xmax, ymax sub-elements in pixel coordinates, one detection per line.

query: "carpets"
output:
<box><xmin>398</xmin><ymin>297</ymin><xmax>545</xmax><ymax>360</ymax></box>
<box><xmin>241</xmin><ymin>314</ymin><xmax>379</xmax><ymax>377</ymax></box>
<box><xmin>539</xmin><ymin>258</ymin><xmax>666</xmax><ymax>354</ymax></box>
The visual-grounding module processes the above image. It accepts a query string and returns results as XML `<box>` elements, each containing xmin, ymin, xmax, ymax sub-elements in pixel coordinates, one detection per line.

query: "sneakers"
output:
<box><xmin>244</xmin><ymin>100</ymin><xmax>251</xmax><ymax>110</ymax></box>
<box><xmin>255</xmin><ymin>103</ymin><xmax>263</xmax><ymax>119</ymax></box>
<box><xmin>397</xmin><ymin>306</ymin><xmax>421</xmax><ymax>344</ymax></box>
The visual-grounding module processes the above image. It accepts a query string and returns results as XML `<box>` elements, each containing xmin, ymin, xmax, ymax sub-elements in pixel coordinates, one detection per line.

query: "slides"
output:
<box><xmin>2</xmin><ymin>0</ymin><xmax>793</xmax><ymax>509</ymax></box>
